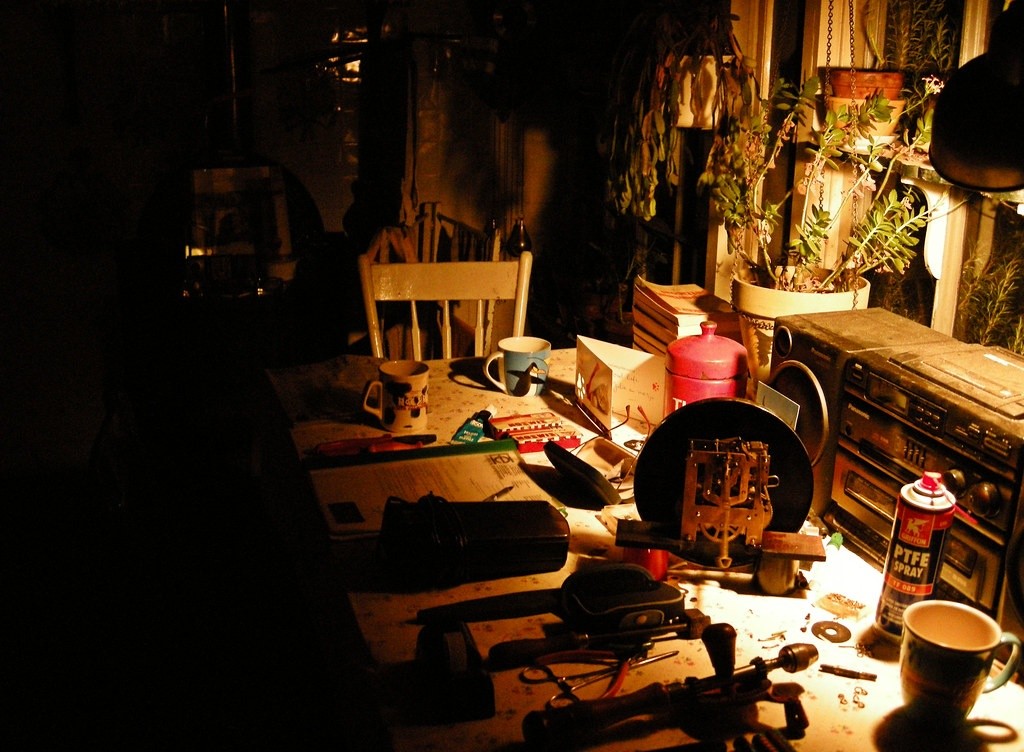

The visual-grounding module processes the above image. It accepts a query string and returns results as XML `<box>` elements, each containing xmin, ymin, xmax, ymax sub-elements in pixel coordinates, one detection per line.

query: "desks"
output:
<box><xmin>259</xmin><ymin>345</ymin><xmax>1024</xmax><ymax>752</ymax></box>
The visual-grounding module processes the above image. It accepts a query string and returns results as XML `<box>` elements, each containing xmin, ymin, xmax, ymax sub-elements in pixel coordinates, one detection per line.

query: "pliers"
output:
<box><xmin>312</xmin><ymin>433</ymin><xmax>436</xmax><ymax>456</ymax></box>
<box><xmin>537</xmin><ymin>641</ymin><xmax>654</xmax><ymax>713</ymax></box>
<box><xmin>753</xmin><ymin>728</ymin><xmax>795</xmax><ymax>752</ymax></box>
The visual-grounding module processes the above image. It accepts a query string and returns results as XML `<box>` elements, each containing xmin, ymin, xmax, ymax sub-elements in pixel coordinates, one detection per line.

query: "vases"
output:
<box><xmin>816</xmin><ymin>67</ymin><xmax>906</xmax><ymax>98</ymax></box>
<box><xmin>820</xmin><ymin>94</ymin><xmax>906</xmax><ymax>141</ymax></box>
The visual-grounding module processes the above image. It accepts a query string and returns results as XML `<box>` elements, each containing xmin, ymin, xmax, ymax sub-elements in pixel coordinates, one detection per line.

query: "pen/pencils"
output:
<box><xmin>819</xmin><ymin>664</ymin><xmax>878</xmax><ymax>681</ymax></box>
<box><xmin>481</xmin><ymin>486</ymin><xmax>514</xmax><ymax>503</ymax></box>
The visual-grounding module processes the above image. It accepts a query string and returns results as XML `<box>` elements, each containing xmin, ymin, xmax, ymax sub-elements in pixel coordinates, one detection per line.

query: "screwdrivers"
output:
<box><xmin>366</xmin><ymin>440</ymin><xmax>452</xmax><ymax>453</ymax></box>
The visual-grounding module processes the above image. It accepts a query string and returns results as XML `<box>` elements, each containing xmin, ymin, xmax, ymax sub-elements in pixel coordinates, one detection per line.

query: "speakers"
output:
<box><xmin>769</xmin><ymin>307</ymin><xmax>959</xmax><ymax>514</ymax></box>
<box><xmin>993</xmin><ymin>480</ymin><xmax>1024</xmax><ymax>675</ymax></box>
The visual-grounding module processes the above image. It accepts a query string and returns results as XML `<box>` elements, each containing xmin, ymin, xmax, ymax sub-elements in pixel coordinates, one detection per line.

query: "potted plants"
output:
<box><xmin>697</xmin><ymin>71</ymin><xmax>945</xmax><ymax>388</ymax></box>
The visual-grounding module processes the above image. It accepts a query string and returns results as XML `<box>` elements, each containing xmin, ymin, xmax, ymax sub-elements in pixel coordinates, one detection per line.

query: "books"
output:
<box><xmin>308</xmin><ymin>434</ymin><xmax>566</xmax><ymax>544</ymax></box>
<box><xmin>632</xmin><ymin>276</ymin><xmax>746</xmax><ymax>358</ymax></box>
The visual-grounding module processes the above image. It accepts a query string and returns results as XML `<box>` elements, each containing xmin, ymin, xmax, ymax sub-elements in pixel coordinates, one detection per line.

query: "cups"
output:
<box><xmin>901</xmin><ymin>599</ymin><xmax>1022</xmax><ymax>718</ymax></box>
<box><xmin>483</xmin><ymin>336</ymin><xmax>551</xmax><ymax>397</ymax></box>
<box><xmin>361</xmin><ymin>360</ymin><xmax>429</xmax><ymax>433</ymax></box>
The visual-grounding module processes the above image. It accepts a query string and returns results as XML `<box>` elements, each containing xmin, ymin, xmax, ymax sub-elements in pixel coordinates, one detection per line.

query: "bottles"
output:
<box><xmin>877</xmin><ymin>470</ymin><xmax>956</xmax><ymax>635</ymax></box>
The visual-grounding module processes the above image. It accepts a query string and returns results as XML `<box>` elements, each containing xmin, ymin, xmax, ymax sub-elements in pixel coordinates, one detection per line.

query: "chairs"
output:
<box><xmin>356</xmin><ymin>250</ymin><xmax>533</xmax><ymax>359</ymax></box>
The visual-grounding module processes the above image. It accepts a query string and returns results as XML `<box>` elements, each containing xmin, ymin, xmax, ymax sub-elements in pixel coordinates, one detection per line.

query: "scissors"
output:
<box><xmin>520</xmin><ymin>649</ymin><xmax>680</xmax><ymax>709</ymax></box>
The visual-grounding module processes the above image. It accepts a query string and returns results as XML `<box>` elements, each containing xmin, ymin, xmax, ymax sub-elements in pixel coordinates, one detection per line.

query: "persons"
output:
<box><xmin>247</xmin><ymin>155</ymin><xmax>327</xmax><ymax>262</ymax></box>
<box><xmin>121</xmin><ymin>190</ymin><xmax>213</xmax><ymax>327</ymax></box>
